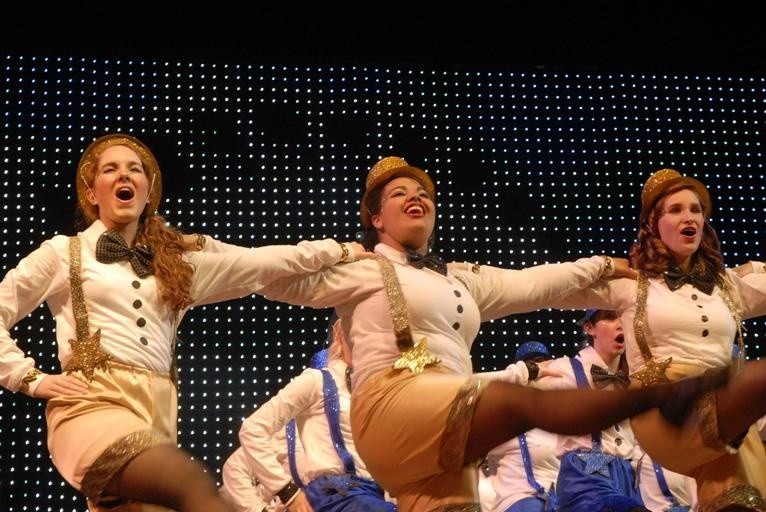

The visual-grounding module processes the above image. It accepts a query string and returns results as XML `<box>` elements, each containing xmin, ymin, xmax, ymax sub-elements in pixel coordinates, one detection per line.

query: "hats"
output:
<box><xmin>76</xmin><ymin>134</ymin><xmax>163</xmax><ymax>219</ymax></box>
<box><xmin>640</xmin><ymin>168</ymin><xmax>712</xmax><ymax>223</ymax></box>
<box><xmin>311</xmin><ymin>349</ymin><xmax>330</xmax><ymax>370</ymax></box>
<box><xmin>360</xmin><ymin>156</ymin><xmax>436</xmax><ymax>224</ymax></box>
<box><xmin>576</xmin><ymin>310</ymin><xmax>597</xmax><ymax>325</ymax></box>
<box><xmin>514</xmin><ymin>341</ymin><xmax>551</xmax><ymax>363</ymax></box>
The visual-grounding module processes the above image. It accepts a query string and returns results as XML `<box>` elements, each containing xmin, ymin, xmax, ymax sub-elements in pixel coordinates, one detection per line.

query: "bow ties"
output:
<box><xmin>664</xmin><ymin>261</ymin><xmax>715</xmax><ymax>295</ymax></box>
<box><xmin>590</xmin><ymin>365</ymin><xmax>630</xmax><ymax>389</ymax></box>
<box><xmin>405</xmin><ymin>245</ymin><xmax>447</xmax><ymax>275</ymax></box>
<box><xmin>96</xmin><ymin>229</ymin><xmax>153</xmax><ymax>278</ymax></box>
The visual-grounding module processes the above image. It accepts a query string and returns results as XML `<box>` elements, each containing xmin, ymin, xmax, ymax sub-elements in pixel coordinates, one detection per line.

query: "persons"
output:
<box><xmin>237</xmin><ymin>311</ymin><xmax>562</xmax><ymax>512</ymax></box>
<box><xmin>631</xmin><ymin>445</ymin><xmax>699</xmax><ymax>511</ymax></box>
<box><xmin>478</xmin><ymin>343</ymin><xmax>568</xmax><ymax>512</ymax></box>
<box><xmin>437</xmin><ymin>169</ymin><xmax>766</xmax><ymax>511</ymax></box>
<box><xmin>216</xmin><ymin>317</ymin><xmax>329</xmax><ymax>511</ymax></box>
<box><xmin>173</xmin><ymin>156</ymin><xmax>733</xmax><ymax>511</ymax></box>
<box><xmin>2</xmin><ymin>134</ymin><xmax>378</xmax><ymax>511</ymax></box>
<box><xmin>523</xmin><ymin>310</ymin><xmax>698</xmax><ymax>510</ymax></box>
